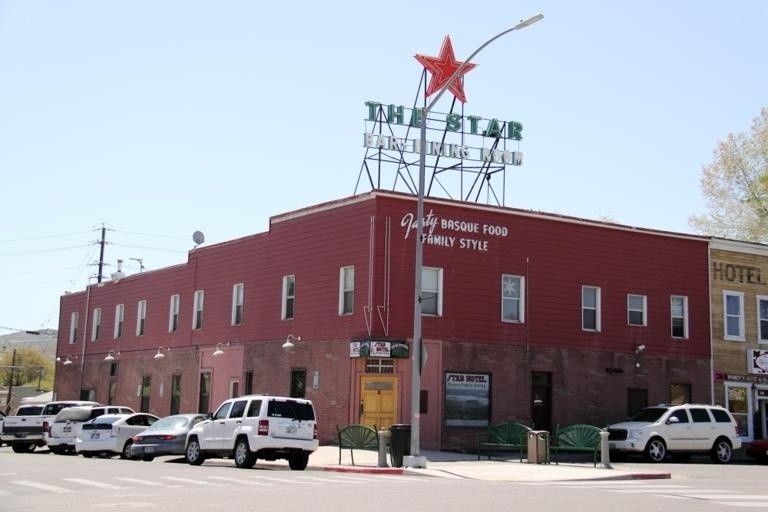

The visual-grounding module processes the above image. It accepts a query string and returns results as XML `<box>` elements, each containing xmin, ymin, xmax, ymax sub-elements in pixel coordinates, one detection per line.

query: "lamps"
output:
<box><xmin>154</xmin><ymin>346</ymin><xmax>170</xmax><ymax>358</ymax></box>
<box><xmin>63</xmin><ymin>354</ymin><xmax>77</xmax><ymax>365</ymax></box>
<box><xmin>105</xmin><ymin>350</ymin><xmax>120</xmax><ymax>361</ymax></box>
<box><xmin>282</xmin><ymin>334</ymin><xmax>302</xmax><ymax>349</ymax></box>
<box><xmin>213</xmin><ymin>341</ymin><xmax>230</xmax><ymax>356</ymax></box>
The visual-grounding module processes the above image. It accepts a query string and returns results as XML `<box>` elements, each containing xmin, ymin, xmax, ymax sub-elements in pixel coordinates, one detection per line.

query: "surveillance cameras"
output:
<box><xmin>637</xmin><ymin>345</ymin><xmax>645</xmax><ymax>351</ymax></box>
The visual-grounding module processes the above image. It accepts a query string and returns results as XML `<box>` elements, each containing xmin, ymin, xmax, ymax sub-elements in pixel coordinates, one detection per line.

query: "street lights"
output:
<box><xmin>402</xmin><ymin>13</ymin><xmax>546</xmax><ymax>468</ymax></box>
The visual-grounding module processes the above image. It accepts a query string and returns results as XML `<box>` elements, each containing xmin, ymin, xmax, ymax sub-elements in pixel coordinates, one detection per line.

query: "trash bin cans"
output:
<box><xmin>527</xmin><ymin>430</ymin><xmax>550</xmax><ymax>463</ymax></box>
<box><xmin>390</xmin><ymin>423</ymin><xmax>411</xmax><ymax>467</ymax></box>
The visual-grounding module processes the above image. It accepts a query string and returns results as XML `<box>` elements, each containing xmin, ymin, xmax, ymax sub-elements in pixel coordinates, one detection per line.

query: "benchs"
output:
<box><xmin>543</xmin><ymin>423</ymin><xmax>609</xmax><ymax>468</ymax></box>
<box><xmin>478</xmin><ymin>421</ymin><xmax>535</xmax><ymax>462</ymax></box>
<box><xmin>335</xmin><ymin>423</ymin><xmax>390</xmax><ymax>466</ymax></box>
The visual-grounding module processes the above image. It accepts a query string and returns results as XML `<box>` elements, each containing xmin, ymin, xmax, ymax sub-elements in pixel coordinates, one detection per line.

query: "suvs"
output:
<box><xmin>184</xmin><ymin>394</ymin><xmax>319</xmax><ymax>469</ymax></box>
<box><xmin>603</xmin><ymin>403</ymin><xmax>742</xmax><ymax>463</ymax></box>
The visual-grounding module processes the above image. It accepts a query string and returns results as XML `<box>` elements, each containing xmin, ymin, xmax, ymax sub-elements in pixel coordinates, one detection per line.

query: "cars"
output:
<box><xmin>131</xmin><ymin>413</ymin><xmax>208</xmax><ymax>461</ymax></box>
<box><xmin>2</xmin><ymin>400</ymin><xmax>159</xmax><ymax>458</ymax></box>
